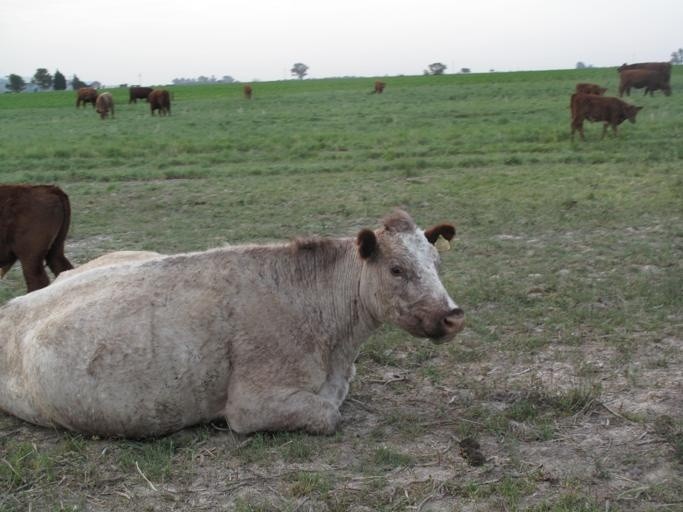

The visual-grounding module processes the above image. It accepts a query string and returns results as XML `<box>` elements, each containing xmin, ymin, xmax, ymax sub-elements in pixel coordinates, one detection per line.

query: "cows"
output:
<box><xmin>618</xmin><ymin>70</ymin><xmax>673</xmax><ymax>97</ymax></box>
<box><xmin>569</xmin><ymin>94</ymin><xmax>644</xmax><ymax>142</ymax></box>
<box><xmin>0</xmin><ymin>184</ymin><xmax>70</xmax><ymax>297</ymax></box>
<box><xmin>244</xmin><ymin>84</ymin><xmax>252</xmax><ymax>98</ymax></box>
<box><xmin>75</xmin><ymin>86</ymin><xmax>97</xmax><ymax>106</ymax></box>
<box><xmin>149</xmin><ymin>89</ymin><xmax>172</xmax><ymax>115</ymax></box>
<box><xmin>126</xmin><ymin>87</ymin><xmax>152</xmax><ymax>103</ymax></box>
<box><xmin>375</xmin><ymin>80</ymin><xmax>385</xmax><ymax>94</ymax></box>
<box><xmin>94</xmin><ymin>91</ymin><xmax>118</xmax><ymax>118</ymax></box>
<box><xmin>621</xmin><ymin>61</ymin><xmax>672</xmax><ymax>71</ymax></box>
<box><xmin>576</xmin><ymin>82</ymin><xmax>607</xmax><ymax>99</ymax></box>
<box><xmin>0</xmin><ymin>209</ymin><xmax>468</xmax><ymax>443</ymax></box>
